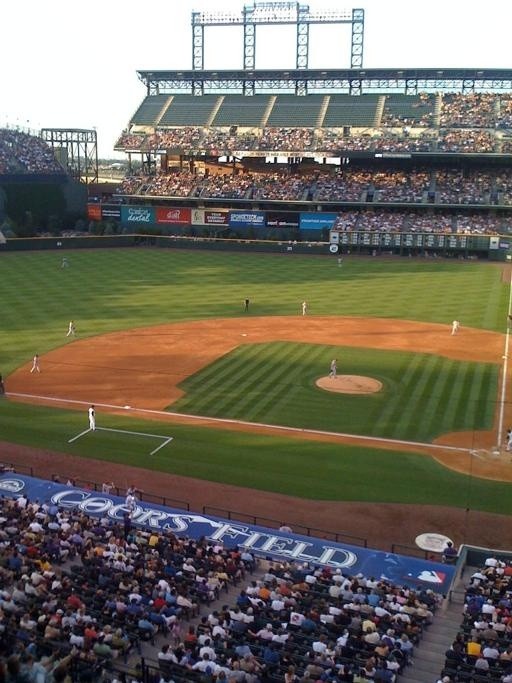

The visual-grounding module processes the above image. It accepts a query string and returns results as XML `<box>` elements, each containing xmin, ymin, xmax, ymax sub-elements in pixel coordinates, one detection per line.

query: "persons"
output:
<box><xmin>244</xmin><ymin>296</ymin><xmax>250</xmax><ymax>311</ymax></box>
<box><xmin>337</xmin><ymin>256</ymin><xmax>344</xmax><ymax>268</ymax></box>
<box><xmin>102</xmin><ymin>480</ymin><xmax>144</xmax><ymax>505</ymax></box>
<box><xmin>328</xmin><ymin>358</ymin><xmax>339</xmax><ymax>379</ymax></box>
<box><xmin>301</xmin><ymin>300</ymin><xmax>309</xmax><ymax>315</ymax></box>
<box><xmin>0</xmin><ymin>494</ymin><xmax>436</xmax><ymax>682</ymax></box>
<box><xmin>443</xmin><ymin>542</ymin><xmax>457</xmax><ymax>559</ymax></box>
<box><xmin>29</xmin><ymin>354</ymin><xmax>41</xmax><ymax>373</ymax></box>
<box><xmin>116</xmin><ymin>87</ymin><xmax>512</xmax><ymax>154</ymax></box>
<box><xmin>505</xmin><ymin>429</ymin><xmax>512</xmax><ymax>452</ymax></box>
<box><xmin>452</xmin><ymin>319</ymin><xmax>460</xmax><ymax>336</ymax></box>
<box><xmin>52</xmin><ymin>474</ymin><xmax>73</xmax><ymax>488</ymax></box>
<box><xmin>66</xmin><ymin>319</ymin><xmax>77</xmax><ymax>337</ymax></box>
<box><xmin>88</xmin><ymin>404</ymin><xmax>96</xmax><ymax>431</ymax></box>
<box><xmin>8</xmin><ymin>464</ymin><xmax>16</xmax><ymax>474</ymax></box>
<box><xmin>116</xmin><ymin>166</ymin><xmax>512</xmax><ymax>237</ymax></box>
<box><xmin>60</xmin><ymin>256</ymin><xmax>70</xmax><ymax>269</ymax></box>
<box><xmin>0</xmin><ymin>373</ymin><xmax>5</xmax><ymax>393</ymax></box>
<box><xmin>438</xmin><ymin>553</ymin><xmax>510</xmax><ymax>682</ymax></box>
<box><xmin>0</xmin><ymin>128</ymin><xmax>61</xmax><ymax>175</ymax></box>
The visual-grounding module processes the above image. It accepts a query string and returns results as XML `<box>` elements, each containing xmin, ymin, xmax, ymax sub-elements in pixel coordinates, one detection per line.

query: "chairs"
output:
<box><xmin>1</xmin><ymin>495</ymin><xmax>512</xmax><ymax>683</ymax></box>
